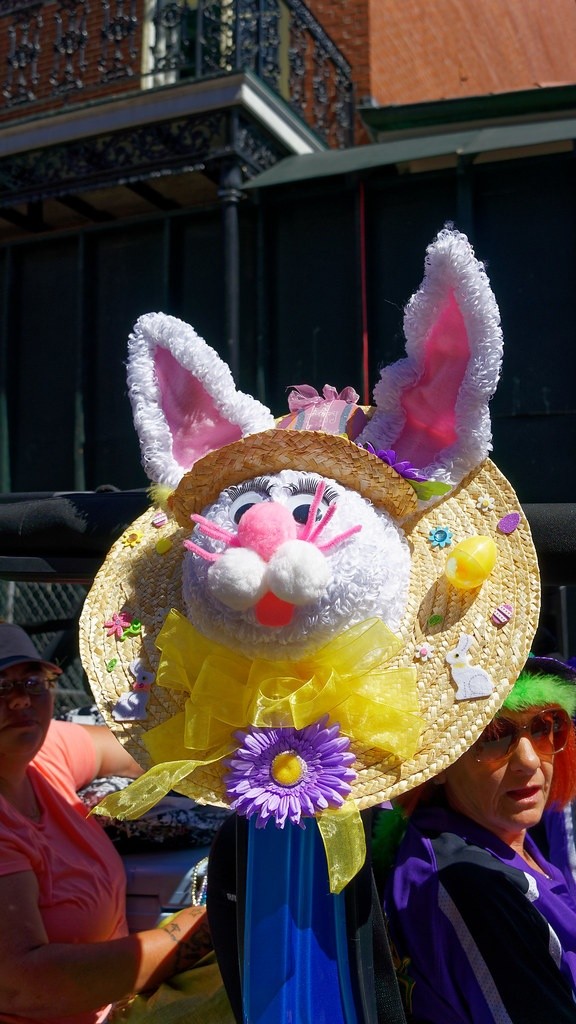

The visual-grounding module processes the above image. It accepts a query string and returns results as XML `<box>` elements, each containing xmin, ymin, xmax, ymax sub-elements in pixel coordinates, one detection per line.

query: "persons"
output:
<box><xmin>0</xmin><ymin>620</ymin><xmax>214</xmax><ymax>1024</ymax></box>
<box><xmin>387</xmin><ymin>654</ymin><xmax>576</xmax><ymax>1022</ymax></box>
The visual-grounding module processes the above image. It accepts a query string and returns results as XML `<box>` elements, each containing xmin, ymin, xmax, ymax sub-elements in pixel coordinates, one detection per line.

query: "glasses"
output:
<box><xmin>0</xmin><ymin>675</ymin><xmax>61</xmax><ymax>694</ymax></box>
<box><xmin>472</xmin><ymin>709</ymin><xmax>570</xmax><ymax>761</ymax></box>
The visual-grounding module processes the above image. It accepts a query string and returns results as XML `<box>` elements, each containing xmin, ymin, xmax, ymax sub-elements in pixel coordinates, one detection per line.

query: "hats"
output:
<box><xmin>0</xmin><ymin>622</ymin><xmax>64</xmax><ymax>673</ymax></box>
<box><xmin>76</xmin><ymin>221</ymin><xmax>541</xmax><ymax>892</ymax></box>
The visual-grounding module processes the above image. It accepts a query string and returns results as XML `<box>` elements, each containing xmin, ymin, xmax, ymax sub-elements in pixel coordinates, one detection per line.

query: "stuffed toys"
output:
<box><xmin>124</xmin><ymin>225</ymin><xmax>503</xmax><ymax>656</ymax></box>
